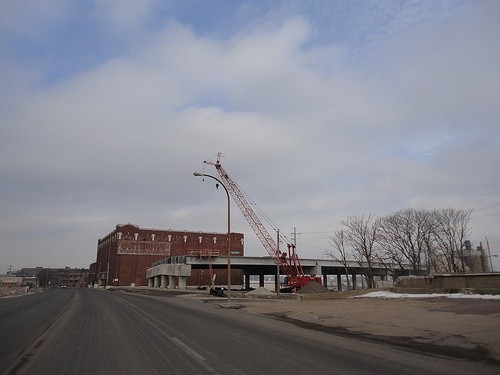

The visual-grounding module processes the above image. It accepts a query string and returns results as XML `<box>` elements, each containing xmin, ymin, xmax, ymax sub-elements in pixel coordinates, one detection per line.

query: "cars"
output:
<box><xmin>210</xmin><ymin>287</ymin><xmax>228</xmax><ymax>298</ymax></box>
<box><xmin>60</xmin><ymin>284</ymin><xmax>66</xmax><ymax>289</ymax></box>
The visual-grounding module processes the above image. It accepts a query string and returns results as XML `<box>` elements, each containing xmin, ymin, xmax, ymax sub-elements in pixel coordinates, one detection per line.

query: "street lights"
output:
<box><xmin>194</xmin><ymin>172</ymin><xmax>232</xmax><ymax>307</ymax></box>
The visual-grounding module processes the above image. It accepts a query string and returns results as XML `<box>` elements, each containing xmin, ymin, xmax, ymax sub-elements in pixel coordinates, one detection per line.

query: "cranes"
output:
<box><xmin>203</xmin><ymin>151</ymin><xmax>322</xmax><ymax>295</ymax></box>
